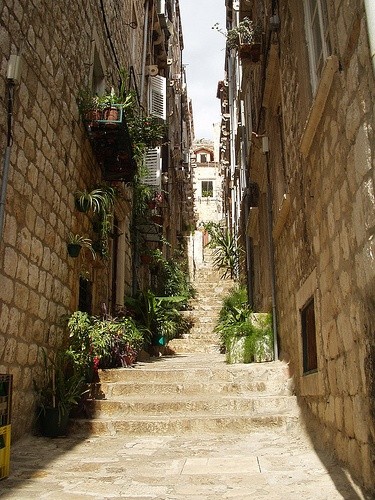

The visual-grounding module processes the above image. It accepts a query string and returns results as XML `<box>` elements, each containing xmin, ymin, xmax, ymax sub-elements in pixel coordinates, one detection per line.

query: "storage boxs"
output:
<box><xmin>0</xmin><ymin>374</ymin><xmax>13</xmax><ymax>427</ymax></box>
<box><xmin>0</xmin><ymin>423</ymin><xmax>11</xmax><ymax>481</ymax></box>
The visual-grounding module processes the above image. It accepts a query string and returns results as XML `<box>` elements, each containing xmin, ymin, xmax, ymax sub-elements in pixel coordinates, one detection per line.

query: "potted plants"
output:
<box><xmin>27</xmin><ymin>16</ymin><xmax>275</xmax><ymax>440</ymax></box>
